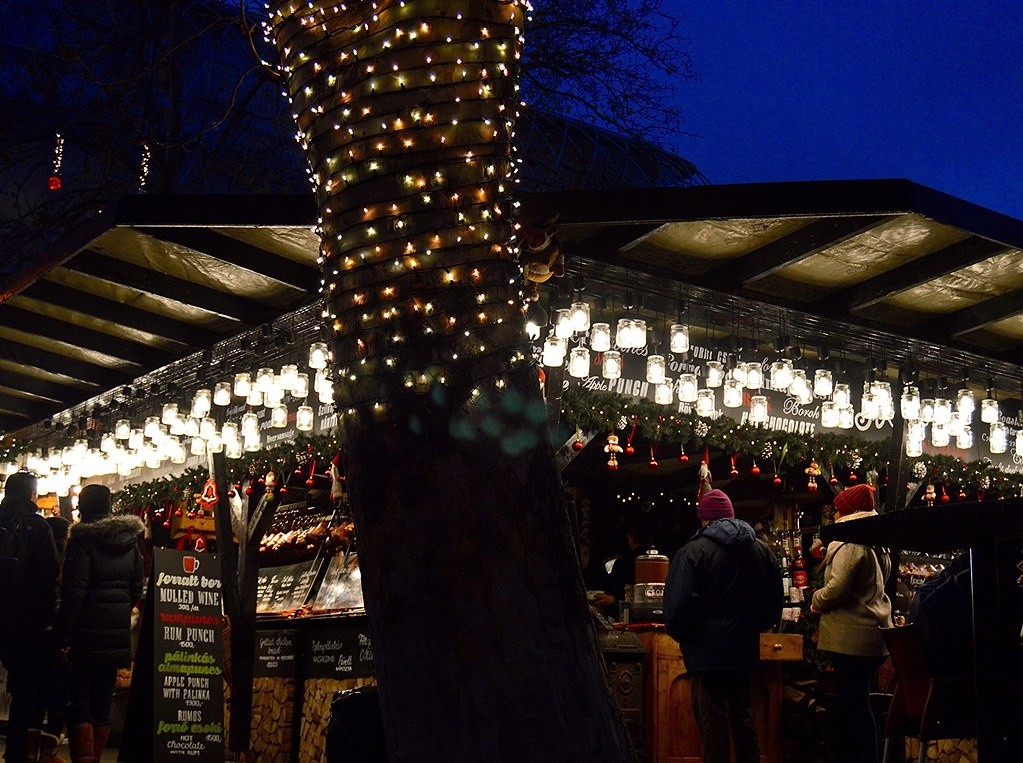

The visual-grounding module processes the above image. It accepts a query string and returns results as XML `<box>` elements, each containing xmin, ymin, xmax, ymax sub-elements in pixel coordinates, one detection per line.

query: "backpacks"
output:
<box><xmin>0</xmin><ymin>511</ymin><xmax>37</xmax><ymax>610</ymax></box>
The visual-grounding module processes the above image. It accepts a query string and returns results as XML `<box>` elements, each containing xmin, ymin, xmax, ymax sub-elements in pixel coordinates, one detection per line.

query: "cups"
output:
<box><xmin>782</xmin><ymin>607</ymin><xmax>801</xmax><ymax>622</ymax></box>
<box><xmin>790</xmin><ymin>586</ymin><xmax>808</xmax><ymax>603</ymax></box>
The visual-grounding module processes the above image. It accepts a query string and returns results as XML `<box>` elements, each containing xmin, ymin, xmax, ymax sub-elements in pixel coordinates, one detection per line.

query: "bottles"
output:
<box><xmin>793</xmin><ymin>531</ymin><xmax>801</xmax><ymax>555</ymax></box>
<box><xmin>775</xmin><ymin>549</ymin><xmax>782</xmax><ymax>566</ymax></box>
<box><xmin>782</xmin><ymin>556</ymin><xmax>792</xmax><ymax>600</ymax></box>
<box><xmin>781</xmin><ymin>519</ymin><xmax>790</xmax><ymax>556</ymax></box>
<box><xmin>795</xmin><ymin>503</ymin><xmax>800</xmax><ymax>529</ymax></box>
<box><xmin>794</xmin><ymin>555</ymin><xmax>807</xmax><ymax>587</ymax></box>
<box><xmin>624</xmin><ymin>608</ymin><xmax>630</xmax><ymax>624</ymax></box>
<box><xmin>897</xmin><ymin>562</ymin><xmax>947</xmax><ymax>577</ymax></box>
<box><xmin>625</xmin><ymin>581</ymin><xmax>632</xmax><ymax>602</ymax></box>
<box><xmin>894</xmin><ymin>609</ymin><xmax>905</xmax><ymax>626</ymax></box>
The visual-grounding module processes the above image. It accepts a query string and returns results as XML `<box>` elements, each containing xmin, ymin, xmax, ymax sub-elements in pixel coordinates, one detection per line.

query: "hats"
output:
<box><xmin>832</xmin><ymin>483</ymin><xmax>874</xmax><ymax>514</ymax></box>
<box><xmin>78</xmin><ymin>485</ymin><xmax>110</xmax><ymax>515</ymax></box>
<box><xmin>697</xmin><ymin>488</ymin><xmax>735</xmax><ymax>520</ymax></box>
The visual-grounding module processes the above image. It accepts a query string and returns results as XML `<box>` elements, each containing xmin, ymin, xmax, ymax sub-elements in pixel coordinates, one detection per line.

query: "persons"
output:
<box><xmin>809</xmin><ymin>483</ymin><xmax>894</xmax><ymax>763</ymax></box>
<box><xmin>905</xmin><ymin>553</ymin><xmax>971</xmax><ymax>622</ymax></box>
<box><xmin>587</xmin><ymin>514</ymin><xmax>693</xmax><ymax>625</ymax></box>
<box><xmin>663</xmin><ymin>489</ymin><xmax>783</xmax><ymax>763</ymax></box>
<box><xmin>0</xmin><ymin>472</ymin><xmax>147</xmax><ymax>763</ymax></box>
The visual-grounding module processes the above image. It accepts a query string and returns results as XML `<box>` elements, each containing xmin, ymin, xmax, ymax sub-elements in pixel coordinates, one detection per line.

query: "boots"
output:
<box><xmin>67</xmin><ymin>722</ymin><xmax>95</xmax><ymax>763</ymax></box>
<box><xmin>24</xmin><ymin>727</ymin><xmax>43</xmax><ymax>763</ymax></box>
<box><xmin>92</xmin><ymin>725</ymin><xmax>111</xmax><ymax>763</ymax></box>
<box><xmin>38</xmin><ymin>732</ymin><xmax>69</xmax><ymax>763</ymax></box>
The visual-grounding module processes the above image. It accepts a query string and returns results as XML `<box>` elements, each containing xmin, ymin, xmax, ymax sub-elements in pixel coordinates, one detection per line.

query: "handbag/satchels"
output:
<box><xmin>37</xmin><ymin>625</ymin><xmax>84</xmax><ymax>697</ymax></box>
